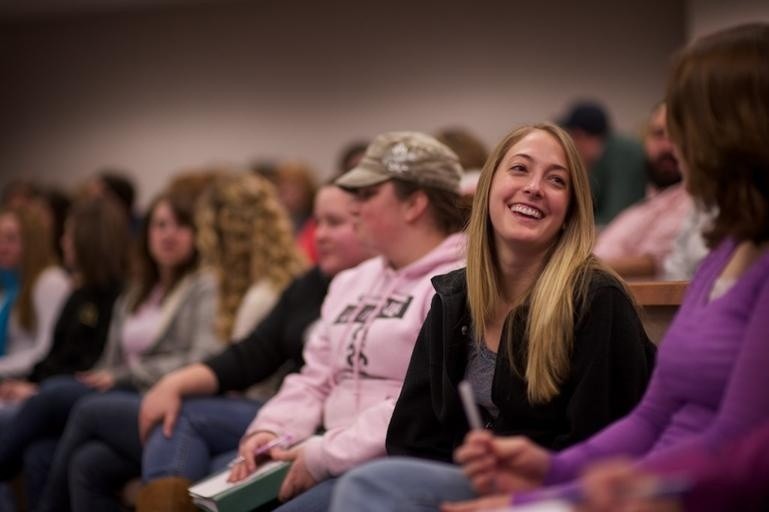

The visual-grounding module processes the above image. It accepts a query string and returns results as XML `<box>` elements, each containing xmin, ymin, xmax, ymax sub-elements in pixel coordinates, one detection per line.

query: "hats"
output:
<box><xmin>333</xmin><ymin>130</ymin><xmax>462</xmax><ymax>194</ymax></box>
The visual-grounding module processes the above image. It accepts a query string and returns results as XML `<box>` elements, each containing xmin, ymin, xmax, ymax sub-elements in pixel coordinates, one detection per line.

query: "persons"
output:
<box><xmin>438</xmin><ymin>21</ymin><xmax>768</xmax><ymax>510</ymax></box>
<box><xmin>228</xmin><ymin>129</ymin><xmax>472</xmax><ymax>512</ymax></box>
<box><xmin>1</xmin><ymin>166</ymin><xmax>377</xmax><ymax>512</ymax></box>
<box><xmin>339</xmin><ymin>100</ymin><xmax>714</xmax><ymax>281</ymax></box>
<box><xmin>328</xmin><ymin>120</ymin><xmax>658</xmax><ymax>512</ymax></box>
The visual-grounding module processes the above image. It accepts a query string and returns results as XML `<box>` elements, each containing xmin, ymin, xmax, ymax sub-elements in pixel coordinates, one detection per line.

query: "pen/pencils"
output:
<box><xmin>455</xmin><ymin>381</ymin><xmax>483</xmax><ymax>431</ymax></box>
<box><xmin>228</xmin><ymin>434</ymin><xmax>293</xmax><ymax>468</ymax></box>
